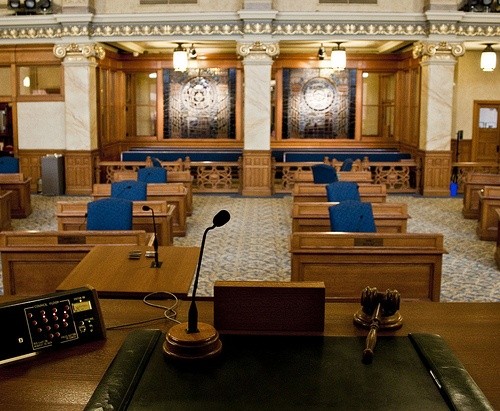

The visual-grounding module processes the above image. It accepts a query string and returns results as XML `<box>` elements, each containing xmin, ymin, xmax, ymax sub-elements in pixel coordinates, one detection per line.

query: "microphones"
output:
<box><xmin>186</xmin><ymin>206</ymin><xmax>231</xmax><ymax>334</ymax></box>
<box><xmin>143</xmin><ymin>205</ymin><xmax>162</xmax><ymax>268</ymax></box>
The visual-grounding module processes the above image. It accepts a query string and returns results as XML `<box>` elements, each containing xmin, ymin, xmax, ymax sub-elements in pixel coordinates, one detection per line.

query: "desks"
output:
<box><xmin>0</xmin><ymin>298</ymin><xmax>500</xmax><ymax>411</ymax></box>
<box><xmin>56</xmin><ymin>247</ymin><xmax>202</xmax><ymax>298</ymax></box>
<box><xmin>452</xmin><ymin>162</ymin><xmax>500</xmax><ymax>193</ymax></box>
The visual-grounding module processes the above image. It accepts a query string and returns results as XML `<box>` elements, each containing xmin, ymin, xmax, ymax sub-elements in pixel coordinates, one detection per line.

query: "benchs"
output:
<box><xmin>293</xmin><ymin>170</ymin><xmax>449</xmax><ymax>301</ymax></box>
<box><xmin>462</xmin><ymin>172</ymin><xmax>500</xmax><ymax>269</ymax></box>
<box><xmin>0</xmin><ymin>170</ymin><xmax>194</xmax><ymax>302</ymax></box>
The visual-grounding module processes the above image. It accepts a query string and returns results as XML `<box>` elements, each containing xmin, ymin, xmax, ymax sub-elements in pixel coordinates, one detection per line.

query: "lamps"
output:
<box><xmin>7</xmin><ymin>0</ymin><xmax>51</xmax><ymax>12</ymax></box>
<box><xmin>331</xmin><ymin>42</ymin><xmax>347</xmax><ymax>70</ymax></box>
<box><xmin>317</xmin><ymin>42</ymin><xmax>324</xmax><ymax>60</ymax></box>
<box><xmin>173</xmin><ymin>43</ymin><xmax>187</xmax><ymax>71</ymax></box>
<box><xmin>190</xmin><ymin>44</ymin><xmax>197</xmax><ymax>60</ymax></box>
<box><xmin>480</xmin><ymin>44</ymin><xmax>497</xmax><ymax>71</ymax></box>
